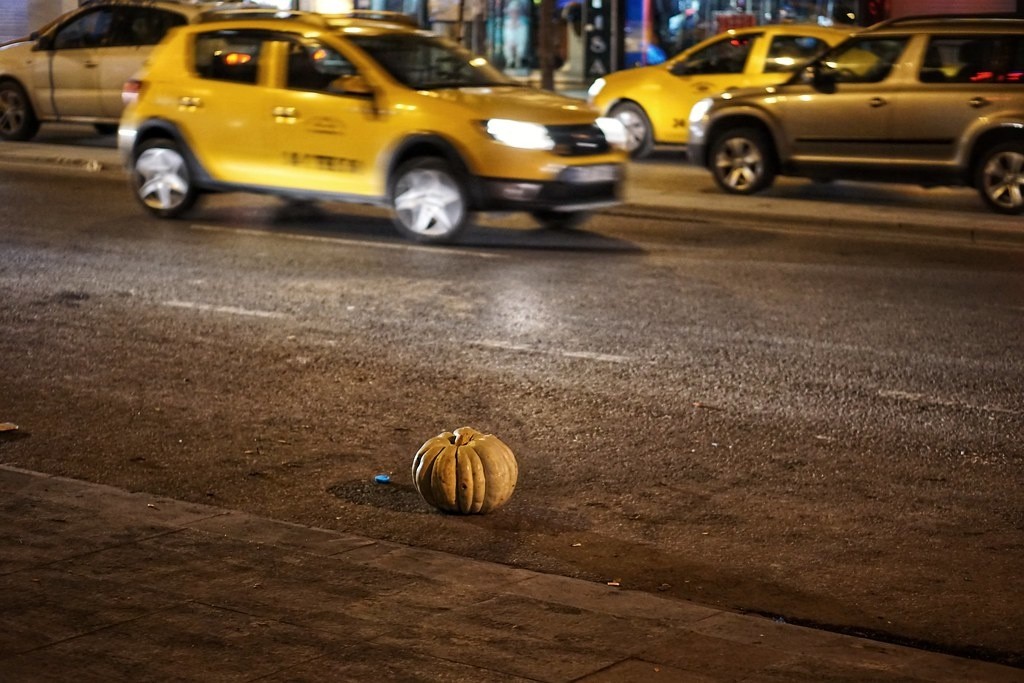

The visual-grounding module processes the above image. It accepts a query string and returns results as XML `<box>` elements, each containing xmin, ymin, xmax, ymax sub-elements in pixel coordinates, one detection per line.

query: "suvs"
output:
<box><xmin>683</xmin><ymin>9</ymin><xmax>1023</xmax><ymax>213</ymax></box>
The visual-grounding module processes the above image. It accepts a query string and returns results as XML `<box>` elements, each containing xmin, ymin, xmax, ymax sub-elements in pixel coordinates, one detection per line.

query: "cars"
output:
<box><xmin>115</xmin><ymin>7</ymin><xmax>634</xmax><ymax>246</ymax></box>
<box><xmin>0</xmin><ymin>1</ymin><xmax>279</xmax><ymax>145</ymax></box>
<box><xmin>585</xmin><ymin>23</ymin><xmax>870</xmax><ymax>165</ymax></box>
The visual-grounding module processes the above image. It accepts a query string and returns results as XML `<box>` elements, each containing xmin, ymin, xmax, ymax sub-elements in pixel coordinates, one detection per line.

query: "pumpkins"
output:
<box><xmin>412</xmin><ymin>426</ymin><xmax>518</xmax><ymax>514</ymax></box>
